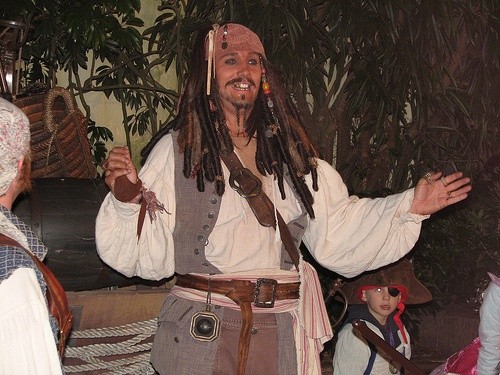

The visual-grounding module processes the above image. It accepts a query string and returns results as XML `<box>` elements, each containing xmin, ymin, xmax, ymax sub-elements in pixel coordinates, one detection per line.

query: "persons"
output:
<box><xmin>326</xmin><ymin>256</ymin><xmax>433</xmax><ymax>375</ymax></box>
<box><xmin>428</xmin><ymin>263</ymin><xmax>500</xmax><ymax>375</ymax></box>
<box><xmin>95</xmin><ymin>22</ymin><xmax>472</xmax><ymax>375</ymax></box>
<box><xmin>0</xmin><ymin>97</ymin><xmax>74</xmax><ymax>375</ymax></box>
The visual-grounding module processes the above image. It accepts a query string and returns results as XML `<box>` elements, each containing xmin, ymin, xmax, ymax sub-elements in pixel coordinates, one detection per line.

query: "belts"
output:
<box><xmin>175</xmin><ymin>274</ymin><xmax>300</xmax><ymax>375</ymax></box>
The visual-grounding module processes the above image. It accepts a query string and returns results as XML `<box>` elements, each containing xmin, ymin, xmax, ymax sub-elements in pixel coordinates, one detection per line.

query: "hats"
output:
<box><xmin>1</xmin><ymin>96</ymin><xmax>31</xmax><ymax>197</ymax></box>
<box><xmin>343</xmin><ymin>257</ymin><xmax>432</xmax><ymax>303</ymax></box>
<box><xmin>204</xmin><ymin>23</ymin><xmax>266</xmax><ymax>96</ymax></box>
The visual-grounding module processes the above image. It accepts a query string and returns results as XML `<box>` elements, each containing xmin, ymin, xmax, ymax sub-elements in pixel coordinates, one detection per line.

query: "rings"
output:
<box><xmin>447</xmin><ymin>191</ymin><xmax>451</xmax><ymax>198</ymax></box>
<box><xmin>440</xmin><ymin>177</ymin><xmax>447</xmax><ymax>187</ymax></box>
<box><xmin>419</xmin><ymin>172</ymin><xmax>432</xmax><ymax>183</ymax></box>
